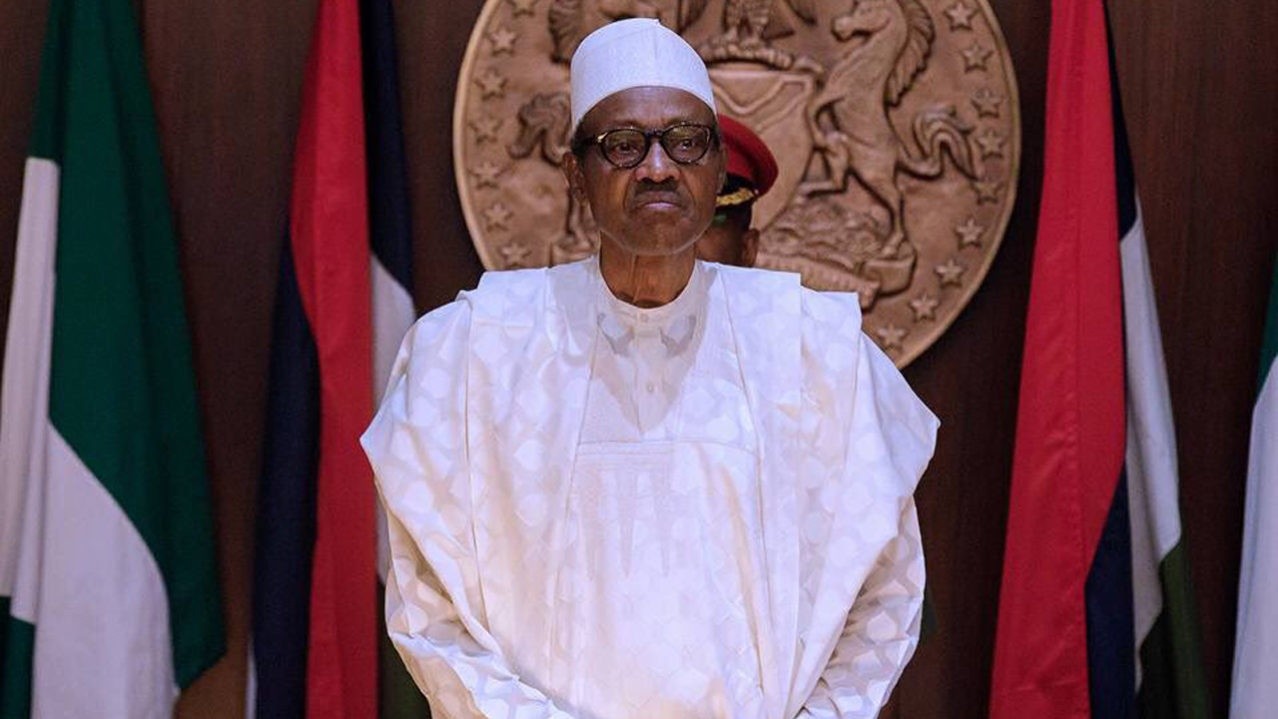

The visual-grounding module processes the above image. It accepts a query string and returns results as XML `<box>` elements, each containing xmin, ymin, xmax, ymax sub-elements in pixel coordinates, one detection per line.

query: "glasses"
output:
<box><xmin>571</xmin><ymin>122</ymin><xmax>714</xmax><ymax>167</ymax></box>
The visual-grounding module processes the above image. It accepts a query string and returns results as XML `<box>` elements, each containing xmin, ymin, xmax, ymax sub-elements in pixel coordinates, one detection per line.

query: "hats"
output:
<box><xmin>571</xmin><ymin>16</ymin><xmax>716</xmax><ymax>142</ymax></box>
<box><xmin>714</xmin><ymin>114</ymin><xmax>779</xmax><ymax>223</ymax></box>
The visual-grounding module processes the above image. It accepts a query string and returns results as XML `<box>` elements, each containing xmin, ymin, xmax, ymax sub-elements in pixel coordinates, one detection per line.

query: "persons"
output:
<box><xmin>376</xmin><ymin>16</ymin><xmax>923</xmax><ymax>718</ymax></box>
<box><xmin>692</xmin><ymin>110</ymin><xmax>779</xmax><ymax>267</ymax></box>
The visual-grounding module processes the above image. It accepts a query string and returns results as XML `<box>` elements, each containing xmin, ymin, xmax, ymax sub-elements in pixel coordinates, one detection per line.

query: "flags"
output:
<box><xmin>244</xmin><ymin>4</ymin><xmax>415</xmax><ymax>717</ymax></box>
<box><xmin>984</xmin><ymin>4</ymin><xmax>1211</xmax><ymax>718</ymax></box>
<box><xmin>0</xmin><ymin>2</ymin><xmax>228</xmax><ymax>718</ymax></box>
